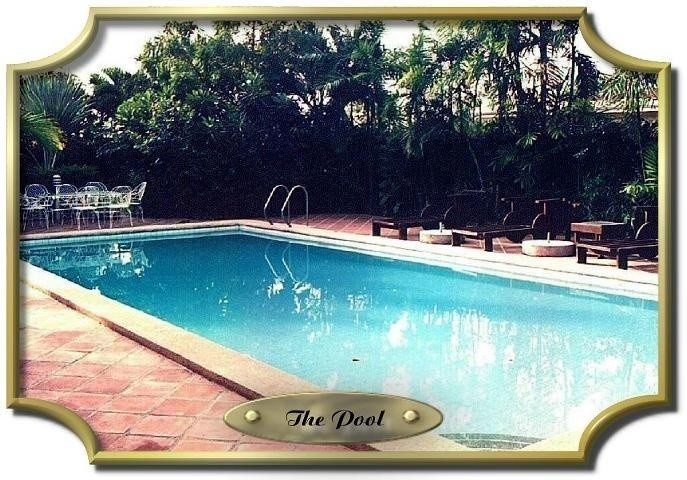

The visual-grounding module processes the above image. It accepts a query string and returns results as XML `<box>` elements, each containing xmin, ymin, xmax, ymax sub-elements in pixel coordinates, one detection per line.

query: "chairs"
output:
<box><xmin>372</xmin><ymin>189</ymin><xmax>658</xmax><ymax>269</ymax></box>
<box><xmin>20</xmin><ymin>180</ymin><xmax>147</xmax><ymax>230</ymax></box>
